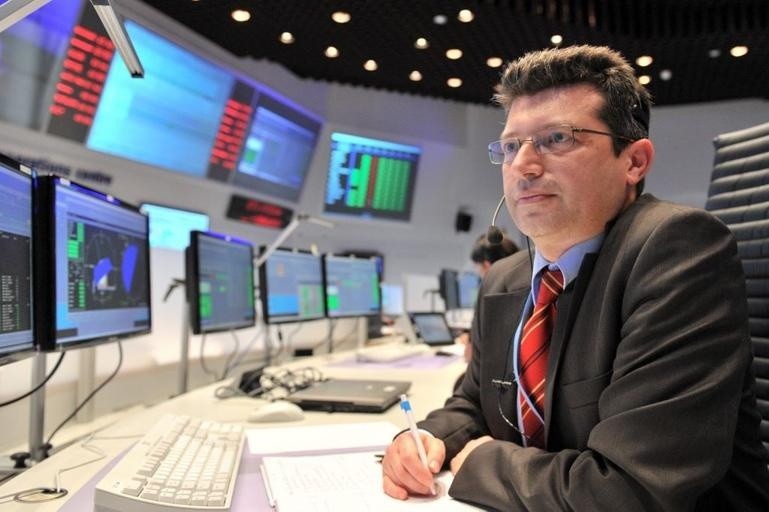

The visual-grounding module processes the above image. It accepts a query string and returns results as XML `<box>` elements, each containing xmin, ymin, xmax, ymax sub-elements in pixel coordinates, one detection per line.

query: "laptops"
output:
<box><xmin>286</xmin><ymin>379</ymin><xmax>412</xmax><ymax>412</ymax></box>
<box><xmin>407</xmin><ymin>311</ymin><xmax>465</xmax><ymax>356</ymax></box>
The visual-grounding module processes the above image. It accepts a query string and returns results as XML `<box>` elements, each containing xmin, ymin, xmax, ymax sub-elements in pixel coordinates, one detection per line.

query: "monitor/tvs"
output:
<box><xmin>34</xmin><ymin>174</ymin><xmax>153</xmax><ymax>352</ymax></box>
<box><xmin>260</xmin><ymin>245</ymin><xmax>326</xmax><ymax>324</ymax></box>
<box><xmin>186</xmin><ymin>230</ymin><xmax>256</xmax><ymax>335</ymax></box>
<box><xmin>439</xmin><ymin>269</ymin><xmax>460</xmax><ymax>310</ymax></box>
<box><xmin>321</xmin><ymin>129</ymin><xmax>426</xmax><ymax>225</ymax></box>
<box><xmin>0</xmin><ymin>0</ymin><xmax>327</xmax><ymax>204</ymax></box>
<box><xmin>459</xmin><ymin>271</ymin><xmax>481</xmax><ymax>306</ymax></box>
<box><xmin>0</xmin><ymin>153</ymin><xmax>38</xmax><ymax>366</ymax></box>
<box><xmin>322</xmin><ymin>254</ymin><xmax>383</xmax><ymax>318</ymax></box>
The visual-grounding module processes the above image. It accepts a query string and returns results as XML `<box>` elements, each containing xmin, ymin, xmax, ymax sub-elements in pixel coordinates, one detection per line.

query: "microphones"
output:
<box><xmin>488</xmin><ymin>196</ymin><xmax>506</xmax><ymax>243</ymax></box>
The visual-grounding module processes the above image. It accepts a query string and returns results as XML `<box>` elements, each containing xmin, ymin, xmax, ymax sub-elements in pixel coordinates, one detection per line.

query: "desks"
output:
<box><xmin>0</xmin><ymin>333</ymin><xmax>492</xmax><ymax>512</ymax></box>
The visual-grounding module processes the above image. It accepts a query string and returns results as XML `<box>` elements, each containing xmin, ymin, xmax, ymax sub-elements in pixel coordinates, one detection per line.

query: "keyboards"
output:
<box><xmin>94</xmin><ymin>417</ymin><xmax>245</xmax><ymax>512</ymax></box>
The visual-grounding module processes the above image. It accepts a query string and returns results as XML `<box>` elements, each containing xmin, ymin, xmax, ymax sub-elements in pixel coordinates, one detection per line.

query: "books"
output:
<box><xmin>260</xmin><ymin>453</ymin><xmax>483</xmax><ymax>511</ymax></box>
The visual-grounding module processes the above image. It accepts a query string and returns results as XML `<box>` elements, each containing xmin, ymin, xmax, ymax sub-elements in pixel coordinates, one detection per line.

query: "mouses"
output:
<box><xmin>246</xmin><ymin>402</ymin><xmax>304</xmax><ymax>423</ymax></box>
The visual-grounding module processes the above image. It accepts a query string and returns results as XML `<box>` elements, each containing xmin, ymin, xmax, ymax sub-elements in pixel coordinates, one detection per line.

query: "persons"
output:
<box><xmin>381</xmin><ymin>45</ymin><xmax>754</xmax><ymax>511</ymax></box>
<box><xmin>461</xmin><ymin>234</ymin><xmax>520</xmax><ymax>362</ymax></box>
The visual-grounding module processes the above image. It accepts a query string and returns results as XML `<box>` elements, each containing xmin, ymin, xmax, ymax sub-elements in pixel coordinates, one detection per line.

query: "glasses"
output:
<box><xmin>486</xmin><ymin>124</ymin><xmax>634</xmax><ymax>165</ymax></box>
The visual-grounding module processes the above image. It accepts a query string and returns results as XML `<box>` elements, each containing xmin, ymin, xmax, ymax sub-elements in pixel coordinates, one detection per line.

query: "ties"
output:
<box><xmin>515</xmin><ymin>266</ymin><xmax>564</xmax><ymax>450</ymax></box>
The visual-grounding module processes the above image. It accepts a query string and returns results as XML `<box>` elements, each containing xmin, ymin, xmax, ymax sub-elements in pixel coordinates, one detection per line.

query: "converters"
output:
<box><xmin>295</xmin><ymin>349</ymin><xmax>313</xmax><ymax>357</ymax></box>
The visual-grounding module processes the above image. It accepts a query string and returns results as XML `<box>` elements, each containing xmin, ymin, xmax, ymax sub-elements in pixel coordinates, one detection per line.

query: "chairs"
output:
<box><xmin>705</xmin><ymin>115</ymin><xmax>768</xmax><ymax>511</ymax></box>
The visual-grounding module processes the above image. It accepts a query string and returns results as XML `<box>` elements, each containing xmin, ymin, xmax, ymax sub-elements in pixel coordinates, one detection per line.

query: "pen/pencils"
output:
<box><xmin>400</xmin><ymin>393</ymin><xmax>437</xmax><ymax>496</ymax></box>
<box><xmin>258</xmin><ymin>465</ymin><xmax>276</xmax><ymax>508</ymax></box>
